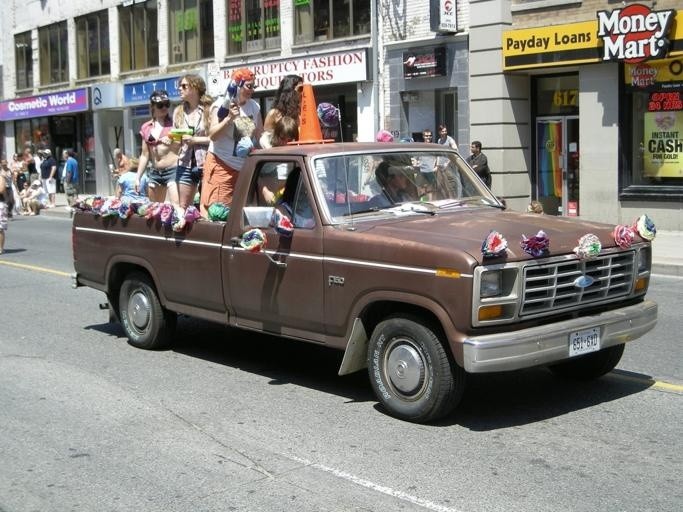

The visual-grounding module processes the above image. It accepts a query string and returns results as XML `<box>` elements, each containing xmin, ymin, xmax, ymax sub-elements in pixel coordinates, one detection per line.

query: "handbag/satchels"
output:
<box><xmin>67</xmin><ymin>186</ymin><xmax>75</xmax><ymax>196</ymax></box>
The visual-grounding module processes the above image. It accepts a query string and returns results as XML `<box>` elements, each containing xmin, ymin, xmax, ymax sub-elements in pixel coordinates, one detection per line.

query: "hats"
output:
<box><xmin>398</xmin><ymin>137</ymin><xmax>415</xmax><ymax>143</ymax></box>
<box><xmin>316</xmin><ymin>103</ymin><xmax>340</xmax><ymax>128</ymax></box>
<box><xmin>30</xmin><ymin>180</ymin><xmax>43</xmax><ymax>186</ymax></box>
<box><xmin>151</xmin><ymin>92</ymin><xmax>171</xmax><ymax>103</ymax></box>
<box><xmin>43</xmin><ymin>149</ymin><xmax>51</xmax><ymax>156</ymax></box>
<box><xmin>376</xmin><ymin>130</ymin><xmax>393</xmax><ymax>142</ymax></box>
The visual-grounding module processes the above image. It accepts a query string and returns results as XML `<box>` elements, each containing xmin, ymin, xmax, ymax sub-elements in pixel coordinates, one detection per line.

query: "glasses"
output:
<box><xmin>177</xmin><ymin>83</ymin><xmax>192</xmax><ymax>89</ymax></box>
<box><xmin>244</xmin><ymin>83</ymin><xmax>258</xmax><ymax>89</ymax></box>
<box><xmin>296</xmin><ymin>86</ymin><xmax>303</xmax><ymax>93</ymax></box>
<box><xmin>155</xmin><ymin>101</ymin><xmax>171</xmax><ymax>110</ymax></box>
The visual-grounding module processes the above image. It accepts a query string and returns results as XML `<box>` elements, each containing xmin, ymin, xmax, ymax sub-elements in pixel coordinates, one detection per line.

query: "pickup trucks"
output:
<box><xmin>69</xmin><ymin>138</ymin><xmax>665</xmax><ymax>427</ymax></box>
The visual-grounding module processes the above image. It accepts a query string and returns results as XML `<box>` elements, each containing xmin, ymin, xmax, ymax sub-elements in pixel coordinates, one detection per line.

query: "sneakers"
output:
<box><xmin>22</xmin><ymin>203</ymin><xmax>71</xmax><ymax>216</ymax></box>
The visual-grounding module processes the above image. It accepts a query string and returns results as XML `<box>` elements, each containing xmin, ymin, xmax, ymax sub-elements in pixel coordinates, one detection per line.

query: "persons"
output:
<box><xmin>108</xmin><ymin>68</ymin><xmax>328</xmax><ymax>230</ymax></box>
<box><xmin>367</xmin><ymin>161</ymin><xmax>414</xmax><ymax>211</ymax></box>
<box><xmin>362</xmin><ymin>124</ymin><xmax>488</xmax><ymax>199</ymax></box>
<box><xmin>0</xmin><ymin>143</ymin><xmax>79</xmax><ymax>255</ymax></box>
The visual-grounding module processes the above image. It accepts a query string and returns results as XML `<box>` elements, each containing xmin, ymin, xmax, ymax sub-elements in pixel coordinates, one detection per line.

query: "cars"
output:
<box><xmin>53</xmin><ymin>157</ymin><xmax>96</xmax><ymax>180</ymax></box>
